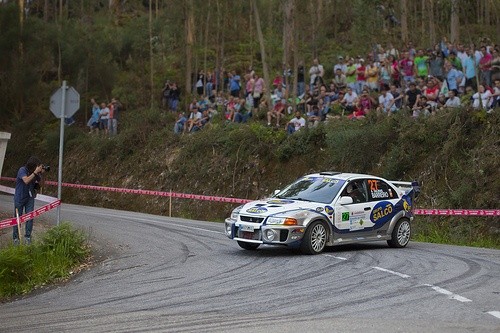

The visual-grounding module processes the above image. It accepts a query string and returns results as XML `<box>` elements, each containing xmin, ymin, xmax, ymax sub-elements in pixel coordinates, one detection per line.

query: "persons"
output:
<box><xmin>227</xmin><ymin>96</ymin><xmax>251</xmax><ymax>123</ymax></box>
<box><xmin>307</xmin><ymin>106</ymin><xmax>324</xmax><ymax>130</ymax></box>
<box><xmin>285</xmin><ymin>112</ymin><xmax>307</xmax><ymax>136</ymax></box>
<box><xmin>175</xmin><ymin>114</ymin><xmax>187</xmax><ymax>133</ymax></box>
<box><xmin>161</xmin><ymin>80</ymin><xmax>180</xmax><ymax>112</ymax></box>
<box><xmin>245</xmin><ymin>74</ymin><xmax>272</xmax><ymax>114</ymax></box>
<box><xmin>188</xmin><ymin>89</ymin><xmax>227</xmax><ymax>134</ymax></box>
<box><xmin>267</xmin><ymin>99</ymin><xmax>285</xmax><ymax>127</ymax></box>
<box><xmin>13</xmin><ymin>156</ymin><xmax>43</xmax><ymax>246</ymax></box>
<box><xmin>191</xmin><ymin>66</ymin><xmax>229</xmax><ymax>98</ymax></box>
<box><xmin>272</xmin><ymin>59</ymin><xmax>339</xmax><ymax>118</ymax></box>
<box><xmin>228</xmin><ymin>71</ymin><xmax>241</xmax><ymax>96</ymax></box>
<box><xmin>334</xmin><ymin>40</ymin><xmax>500</xmax><ymax>120</ymax></box>
<box><xmin>87</xmin><ymin>98</ymin><xmax>122</xmax><ymax>140</ymax></box>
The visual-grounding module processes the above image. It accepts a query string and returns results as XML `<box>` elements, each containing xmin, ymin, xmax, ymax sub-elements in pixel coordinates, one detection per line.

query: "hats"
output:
<box><xmin>275</xmin><ymin>97</ymin><xmax>281</xmax><ymax>102</ymax></box>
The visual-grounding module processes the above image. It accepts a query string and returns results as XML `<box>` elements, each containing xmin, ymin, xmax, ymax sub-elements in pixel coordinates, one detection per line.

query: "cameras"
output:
<box><xmin>41</xmin><ymin>163</ymin><xmax>51</xmax><ymax>172</ymax></box>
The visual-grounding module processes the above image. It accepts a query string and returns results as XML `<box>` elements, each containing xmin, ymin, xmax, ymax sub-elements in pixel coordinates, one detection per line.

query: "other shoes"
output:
<box><xmin>486</xmin><ymin>109</ymin><xmax>495</xmax><ymax>113</ymax></box>
<box><xmin>24</xmin><ymin>237</ymin><xmax>31</xmax><ymax>245</ymax></box>
<box><xmin>14</xmin><ymin>239</ymin><xmax>19</xmax><ymax>246</ymax></box>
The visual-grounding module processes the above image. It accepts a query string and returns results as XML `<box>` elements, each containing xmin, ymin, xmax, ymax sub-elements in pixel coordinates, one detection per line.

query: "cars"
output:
<box><xmin>224</xmin><ymin>171</ymin><xmax>421</xmax><ymax>256</ymax></box>
